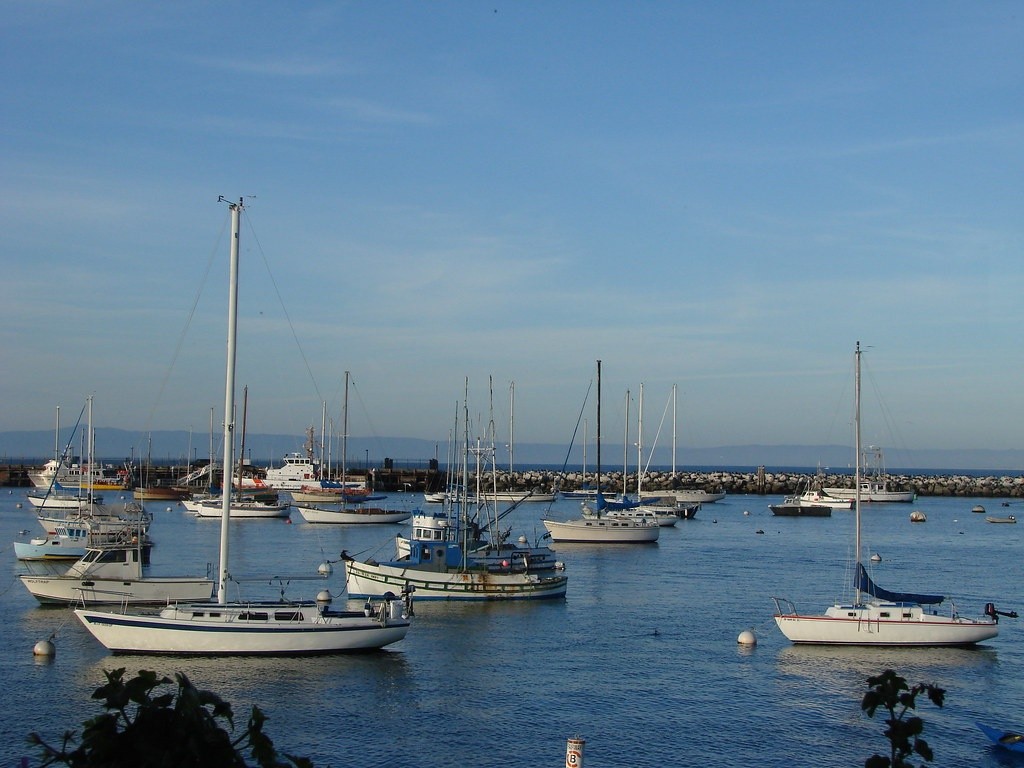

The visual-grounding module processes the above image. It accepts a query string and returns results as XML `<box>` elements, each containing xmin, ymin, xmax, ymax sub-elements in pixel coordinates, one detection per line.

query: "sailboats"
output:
<box><xmin>26</xmin><ymin>380</ymin><xmax>410</xmax><ymax>533</ymax></box>
<box><xmin>540</xmin><ymin>357</ymin><xmax>661</xmax><ymax>544</ymax></box>
<box><xmin>344</xmin><ymin>376</ymin><xmax>570</xmax><ymax>603</ymax></box>
<box><xmin>70</xmin><ymin>191</ymin><xmax>413</xmax><ymax>652</ymax></box>
<box><xmin>770</xmin><ymin>339</ymin><xmax>1020</xmax><ymax>646</ymax></box>
<box><xmin>580</xmin><ymin>380</ymin><xmax>728</xmax><ymax>526</ymax></box>
<box><xmin>14</xmin><ymin>397</ymin><xmax>157</xmax><ymax>569</ymax></box>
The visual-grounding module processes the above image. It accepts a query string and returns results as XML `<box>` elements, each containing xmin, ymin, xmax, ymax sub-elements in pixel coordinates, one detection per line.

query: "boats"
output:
<box><xmin>784</xmin><ymin>463</ymin><xmax>855</xmax><ymax>511</ymax></box>
<box><xmin>819</xmin><ymin>444</ymin><xmax>915</xmax><ymax>503</ymax></box>
<box><xmin>768</xmin><ymin>473</ymin><xmax>833</xmax><ymax>518</ymax></box>
<box><xmin>19</xmin><ymin>395</ymin><xmax>216</xmax><ymax>608</ymax></box>
